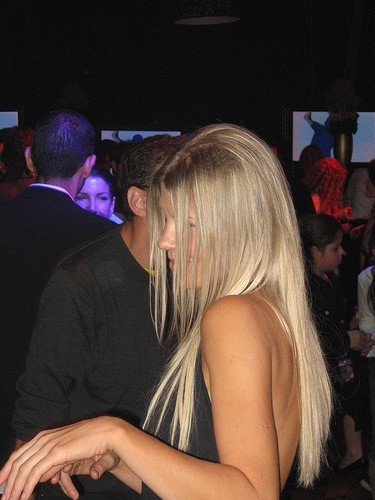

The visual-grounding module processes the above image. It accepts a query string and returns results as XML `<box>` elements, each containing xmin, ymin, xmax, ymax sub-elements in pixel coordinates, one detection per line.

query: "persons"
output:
<box><xmin>0</xmin><ymin>122</ymin><xmax>333</xmax><ymax>500</ymax></box>
<box><xmin>345</xmin><ymin>365</ymin><xmax>354</xmax><ymax>379</ymax></box>
<box><xmin>9</xmin><ymin>135</ymin><xmax>182</xmax><ymax>500</ymax></box>
<box><xmin>0</xmin><ymin>109</ymin><xmax>133</xmax><ymax>466</ymax></box>
<box><xmin>298</xmin><ymin>156</ymin><xmax>375</xmax><ymax>500</ymax></box>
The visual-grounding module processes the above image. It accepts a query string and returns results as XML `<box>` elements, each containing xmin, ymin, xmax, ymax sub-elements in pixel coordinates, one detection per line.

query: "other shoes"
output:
<box><xmin>359</xmin><ymin>475</ymin><xmax>372</xmax><ymax>493</ymax></box>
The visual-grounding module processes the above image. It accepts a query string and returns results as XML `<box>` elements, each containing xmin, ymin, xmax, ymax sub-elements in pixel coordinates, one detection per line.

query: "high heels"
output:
<box><xmin>336</xmin><ymin>455</ymin><xmax>369</xmax><ymax>475</ymax></box>
